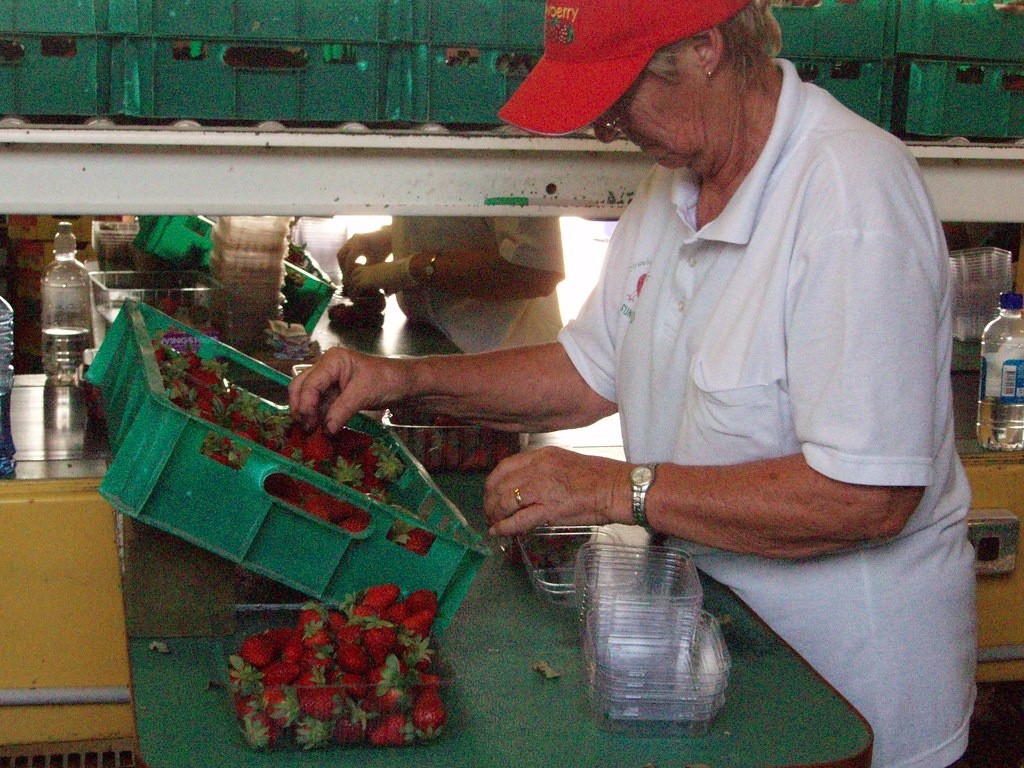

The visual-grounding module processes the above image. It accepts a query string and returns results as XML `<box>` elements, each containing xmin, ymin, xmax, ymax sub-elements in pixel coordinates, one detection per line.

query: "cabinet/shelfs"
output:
<box><xmin>946</xmin><ymin>378</ymin><xmax>1024</xmax><ymax>683</ymax></box>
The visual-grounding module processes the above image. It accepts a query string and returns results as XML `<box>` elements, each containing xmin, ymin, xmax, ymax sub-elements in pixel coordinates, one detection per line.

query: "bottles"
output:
<box><xmin>0</xmin><ymin>296</ymin><xmax>18</xmax><ymax>477</ymax></box>
<box><xmin>39</xmin><ymin>221</ymin><xmax>92</xmax><ymax>385</ymax></box>
<box><xmin>976</xmin><ymin>293</ymin><xmax>1024</xmax><ymax>451</ymax></box>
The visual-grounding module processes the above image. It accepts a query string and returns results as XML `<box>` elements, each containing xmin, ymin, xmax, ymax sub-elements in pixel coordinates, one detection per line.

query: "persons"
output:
<box><xmin>286</xmin><ymin>0</ymin><xmax>979</xmax><ymax>768</ymax></box>
<box><xmin>336</xmin><ymin>215</ymin><xmax>565</xmax><ymax>453</ymax></box>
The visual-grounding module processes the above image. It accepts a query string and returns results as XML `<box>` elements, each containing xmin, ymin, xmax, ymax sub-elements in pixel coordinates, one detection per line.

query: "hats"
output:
<box><xmin>498</xmin><ymin>0</ymin><xmax>752</xmax><ymax>135</ymax></box>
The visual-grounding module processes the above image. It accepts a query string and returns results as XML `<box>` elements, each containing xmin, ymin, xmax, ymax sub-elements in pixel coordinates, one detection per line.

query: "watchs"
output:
<box><xmin>628</xmin><ymin>460</ymin><xmax>658</xmax><ymax>532</ymax></box>
<box><xmin>423</xmin><ymin>252</ymin><xmax>440</xmax><ymax>281</ymax></box>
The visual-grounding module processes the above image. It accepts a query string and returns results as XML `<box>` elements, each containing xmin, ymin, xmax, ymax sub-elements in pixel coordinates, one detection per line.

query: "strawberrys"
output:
<box><xmin>503</xmin><ymin>521</ymin><xmax>579</xmax><ymax>569</ymax></box>
<box><xmin>226</xmin><ymin>585</ymin><xmax>446</xmax><ymax>750</ymax></box>
<box><xmin>387</xmin><ymin>404</ymin><xmax>524</xmax><ymax>476</ymax></box>
<box><xmin>150</xmin><ymin>244</ymin><xmax>439</xmax><ymax>555</ymax></box>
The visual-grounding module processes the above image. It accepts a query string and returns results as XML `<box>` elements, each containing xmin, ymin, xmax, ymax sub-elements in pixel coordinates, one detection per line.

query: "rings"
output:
<box><xmin>513</xmin><ymin>488</ymin><xmax>524</xmax><ymax>507</ymax></box>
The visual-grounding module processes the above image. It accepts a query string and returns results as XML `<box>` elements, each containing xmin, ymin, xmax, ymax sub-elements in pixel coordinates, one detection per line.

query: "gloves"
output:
<box><xmin>337</xmin><ymin>229</ymin><xmax>392</xmax><ymax>272</ymax></box>
<box><xmin>345</xmin><ymin>257</ymin><xmax>416</xmax><ymax>294</ymax></box>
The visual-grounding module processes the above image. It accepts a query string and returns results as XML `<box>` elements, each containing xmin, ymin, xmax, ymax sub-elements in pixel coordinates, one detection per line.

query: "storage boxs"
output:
<box><xmin>133</xmin><ymin>214</ymin><xmax>337</xmax><ymax>338</ymax></box>
<box><xmin>85</xmin><ymin>297</ymin><xmax>490</xmax><ymax>644</ymax></box>
<box><xmin>0</xmin><ymin>0</ymin><xmax>1024</xmax><ymax>139</ymax></box>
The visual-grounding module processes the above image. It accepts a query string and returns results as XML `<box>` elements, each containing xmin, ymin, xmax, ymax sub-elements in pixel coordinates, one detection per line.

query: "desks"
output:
<box><xmin>111</xmin><ymin>291</ymin><xmax>877</xmax><ymax>768</ymax></box>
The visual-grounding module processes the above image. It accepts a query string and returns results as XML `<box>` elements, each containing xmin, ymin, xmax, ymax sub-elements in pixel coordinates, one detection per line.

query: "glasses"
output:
<box><xmin>593</xmin><ymin>82</ymin><xmax>642</xmax><ymax>133</ymax></box>
